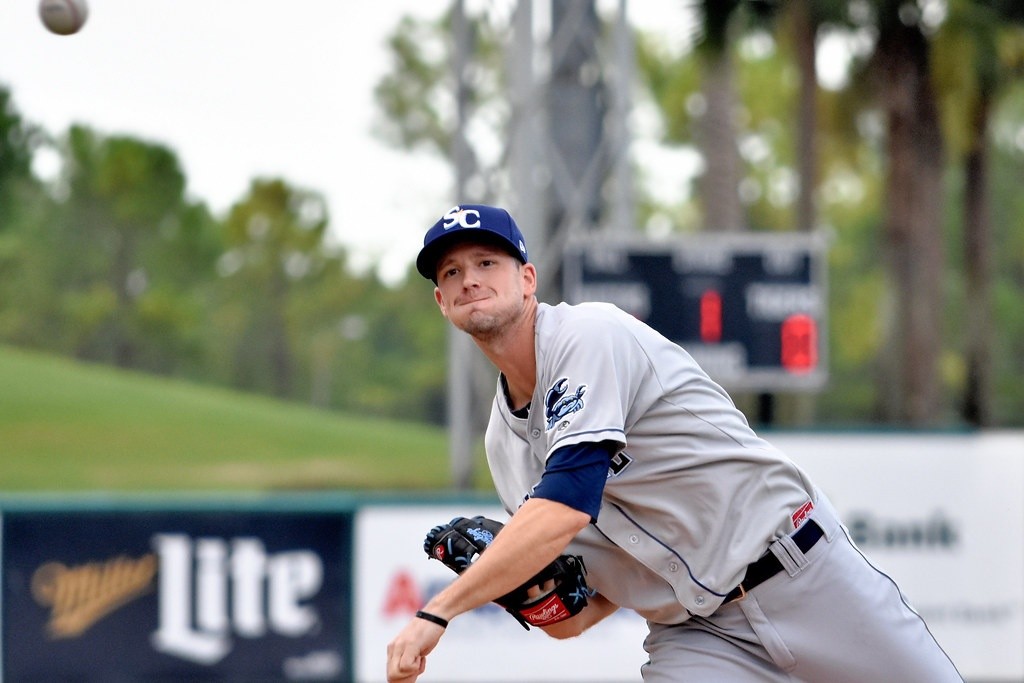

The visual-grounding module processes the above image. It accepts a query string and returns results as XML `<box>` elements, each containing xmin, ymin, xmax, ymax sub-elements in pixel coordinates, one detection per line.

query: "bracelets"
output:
<box><xmin>416</xmin><ymin>610</ymin><xmax>448</xmax><ymax>628</ymax></box>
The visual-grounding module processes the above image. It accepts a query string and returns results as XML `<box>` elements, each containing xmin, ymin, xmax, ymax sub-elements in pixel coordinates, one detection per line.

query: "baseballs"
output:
<box><xmin>37</xmin><ymin>0</ymin><xmax>90</xmax><ymax>36</ymax></box>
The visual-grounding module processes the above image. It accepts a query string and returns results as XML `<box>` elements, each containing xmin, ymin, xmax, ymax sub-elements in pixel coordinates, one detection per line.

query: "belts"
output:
<box><xmin>719</xmin><ymin>519</ymin><xmax>824</xmax><ymax>607</ymax></box>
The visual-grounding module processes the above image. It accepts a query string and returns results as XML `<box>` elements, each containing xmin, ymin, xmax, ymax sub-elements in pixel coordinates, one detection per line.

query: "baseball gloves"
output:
<box><xmin>423</xmin><ymin>512</ymin><xmax>598</xmax><ymax>632</ymax></box>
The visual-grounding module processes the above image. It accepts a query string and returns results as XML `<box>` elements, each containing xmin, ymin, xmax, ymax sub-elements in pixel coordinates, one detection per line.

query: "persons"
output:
<box><xmin>387</xmin><ymin>204</ymin><xmax>967</xmax><ymax>683</ymax></box>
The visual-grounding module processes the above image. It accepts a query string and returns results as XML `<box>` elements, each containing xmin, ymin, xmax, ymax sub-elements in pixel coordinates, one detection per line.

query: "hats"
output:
<box><xmin>416</xmin><ymin>205</ymin><xmax>527</xmax><ymax>286</ymax></box>
